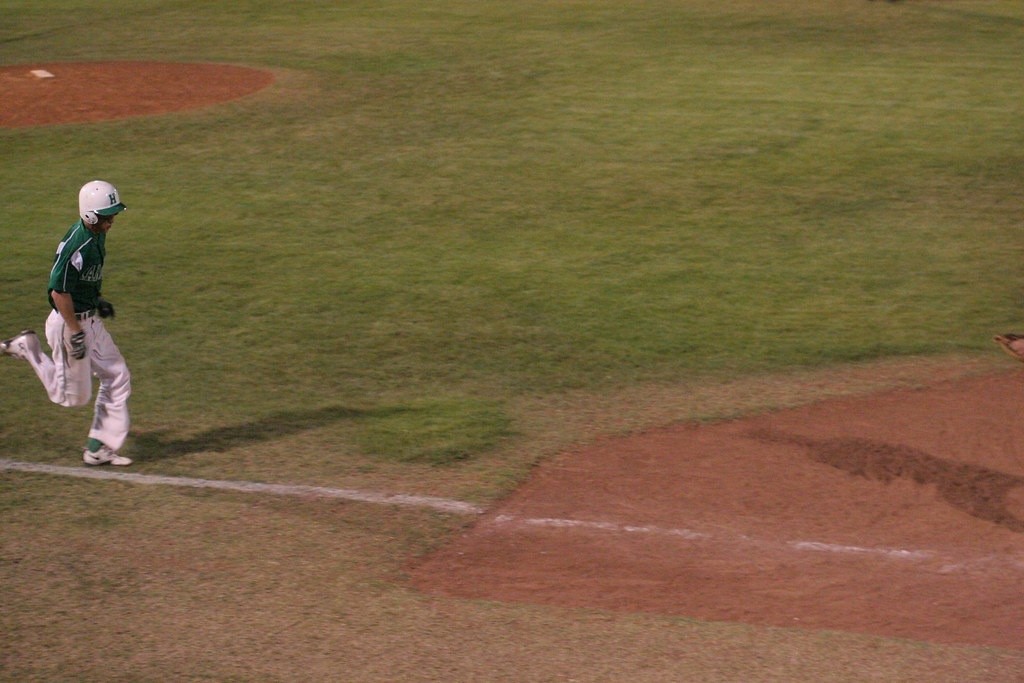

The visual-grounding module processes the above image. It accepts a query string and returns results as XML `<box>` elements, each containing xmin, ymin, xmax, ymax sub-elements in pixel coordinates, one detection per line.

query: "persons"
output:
<box><xmin>0</xmin><ymin>180</ymin><xmax>135</xmax><ymax>466</ymax></box>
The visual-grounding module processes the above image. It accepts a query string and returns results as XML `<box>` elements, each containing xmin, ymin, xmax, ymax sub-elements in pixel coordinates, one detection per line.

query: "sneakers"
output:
<box><xmin>0</xmin><ymin>329</ymin><xmax>40</xmax><ymax>354</ymax></box>
<box><xmin>82</xmin><ymin>445</ymin><xmax>133</xmax><ymax>465</ymax></box>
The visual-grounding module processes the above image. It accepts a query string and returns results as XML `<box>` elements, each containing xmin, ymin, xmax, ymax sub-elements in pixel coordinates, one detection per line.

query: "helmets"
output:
<box><xmin>78</xmin><ymin>180</ymin><xmax>126</xmax><ymax>225</ymax></box>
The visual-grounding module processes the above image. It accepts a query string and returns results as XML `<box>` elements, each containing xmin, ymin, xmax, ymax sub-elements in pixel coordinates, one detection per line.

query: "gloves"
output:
<box><xmin>96</xmin><ymin>301</ymin><xmax>115</xmax><ymax>319</ymax></box>
<box><xmin>70</xmin><ymin>332</ymin><xmax>86</xmax><ymax>359</ymax></box>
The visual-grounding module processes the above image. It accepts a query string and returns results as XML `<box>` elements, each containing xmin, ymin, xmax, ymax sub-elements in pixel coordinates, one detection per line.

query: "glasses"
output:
<box><xmin>99</xmin><ymin>211</ymin><xmax>119</xmax><ymax>221</ymax></box>
